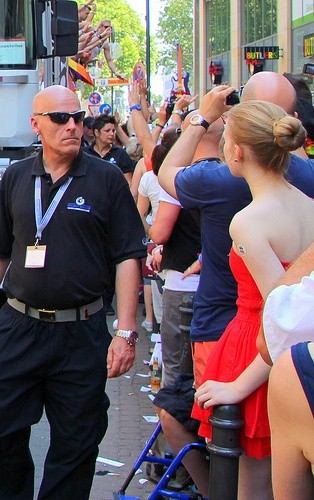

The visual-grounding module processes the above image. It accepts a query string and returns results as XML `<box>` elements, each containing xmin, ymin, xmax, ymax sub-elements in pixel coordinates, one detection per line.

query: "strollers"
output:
<box><xmin>113</xmin><ymin>419</ymin><xmax>209</xmax><ymax>500</ymax></box>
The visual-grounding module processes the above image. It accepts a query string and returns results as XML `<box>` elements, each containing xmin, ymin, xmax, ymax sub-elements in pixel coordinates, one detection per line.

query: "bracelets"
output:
<box><xmin>99</xmin><ymin>40</ymin><xmax>104</xmax><ymax>43</ymax></box>
<box><xmin>96</xmin><ymin>32</ymin><xmax>99</xmax><ymax>36</ymax></box>
<box><xmin>99</xmin><ymin>34</ymin><xmax>103</xmax><ymax>38</ymax></box>
<box><xmin>155</xmin><ymin>123</ymin><xmax>165</xmax><ymax>128</ymax></box>
<box><xmin>97</xmin><ymin>36</ymin><xmax>100</xmax><ymax>39</ymax></box>
<box><xmin>114</xmin><ymin>72</ymin><xmax>119</xmax><ymax>75</ymax></box>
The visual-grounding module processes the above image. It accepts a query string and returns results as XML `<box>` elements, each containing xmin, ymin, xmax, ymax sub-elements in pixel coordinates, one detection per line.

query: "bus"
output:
<box><xmin>0</xmin><ymin>0</ymin><xmax>94</xmax><ymax>182</ymax></box>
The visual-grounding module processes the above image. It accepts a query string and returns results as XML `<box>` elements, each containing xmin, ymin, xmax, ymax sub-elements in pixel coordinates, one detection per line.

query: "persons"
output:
<box><xmin>0</xmin><ymin>0</ymin><xmax>314</xmax><ymax>500</ymax></box>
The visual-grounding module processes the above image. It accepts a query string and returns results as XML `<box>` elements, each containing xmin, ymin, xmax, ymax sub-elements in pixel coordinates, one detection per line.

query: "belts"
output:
<box><xmin>6</xmin><ymin>297</ymin><xmax>103</xmax><ymax>322</ymax></box>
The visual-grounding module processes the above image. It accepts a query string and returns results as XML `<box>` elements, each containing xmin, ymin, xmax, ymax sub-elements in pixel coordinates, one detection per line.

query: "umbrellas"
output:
<box><xmin>68</xmin><ymin>58</ymin><xmax>94</xmax><ymax>86</ymax></box>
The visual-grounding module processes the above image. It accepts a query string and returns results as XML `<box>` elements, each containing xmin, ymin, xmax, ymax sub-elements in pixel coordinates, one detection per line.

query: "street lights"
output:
<box><xmin>105</xmin><ymin>43</ymin><xmax>122</xmax><ymax>117</ymax></box>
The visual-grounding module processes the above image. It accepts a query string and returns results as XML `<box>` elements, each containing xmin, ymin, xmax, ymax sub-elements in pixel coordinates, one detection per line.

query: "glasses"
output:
<box><xmin>86</xmin><ymin>125</ymin><xmax>92</xmax><ymax>129</ymax></box>
<box><xmin>33</xmin><ymin>110</ymin><xmax>85</xmax><ymax>124</ymax></box>
<box><xmin>104</xmin><ymin>26</ymin><xmax>109</xmax><ymax>30</ymax></box>
<box><xmin>239</xmin><ymin>86</ymin><xmax>244</xmax><ymax>98</ymax></box>
<box><xmin>176</xmin><ymin>127</ymin><xmax>182</xmax><ymax>137</ymax></box>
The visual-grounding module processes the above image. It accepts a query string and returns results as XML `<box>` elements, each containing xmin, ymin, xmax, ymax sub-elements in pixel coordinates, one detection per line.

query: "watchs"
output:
<box><xmin>155</xmin><ymin>249</ymin><xmax>163</xmax><ymax>254</ymax></box>
<box><xmin>84</xmin><ymin>3</ymin><xmax>86</xmax><ymax>7</ymax></box>
<box><xmin>129</xmin><ymin>103</ymin><xmax>143</xmax><ymax>112</ymax></box>
<box><xmin>114</xmin><ymin>329</ymin><xmax>139</xmax><ymax>346</ymax></box>
<box><xmin>189</xmin><ymin>114</ymin><xmax>211</xmax><ymax>132</ymax></box>
<box><xmin>94</xmin><ymin>29</ymin><xmax>97</xmax><ymax>33</ymax></box>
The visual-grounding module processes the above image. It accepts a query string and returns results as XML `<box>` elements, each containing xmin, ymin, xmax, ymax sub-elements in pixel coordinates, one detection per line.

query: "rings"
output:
<box><xmin>188</xmin><ymin>267</ymin><xmax>191</xmax><ymax>271</ymax></box>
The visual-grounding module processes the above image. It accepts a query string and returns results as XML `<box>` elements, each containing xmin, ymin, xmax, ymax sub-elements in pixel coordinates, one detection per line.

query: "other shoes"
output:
<box><xmin>167</xmin><ymin>465</ymin><xmax>195</xmax><ymax>490</ymax></box>
<box><xmin>106</xmin><ymin>304</ymin><xmax>115</xmax><ymax>315</ymax></box>
<box><xmin>141</xmin><ymin>320</ymin><xmax>153</xmax><ymax>331</ymax></box>
